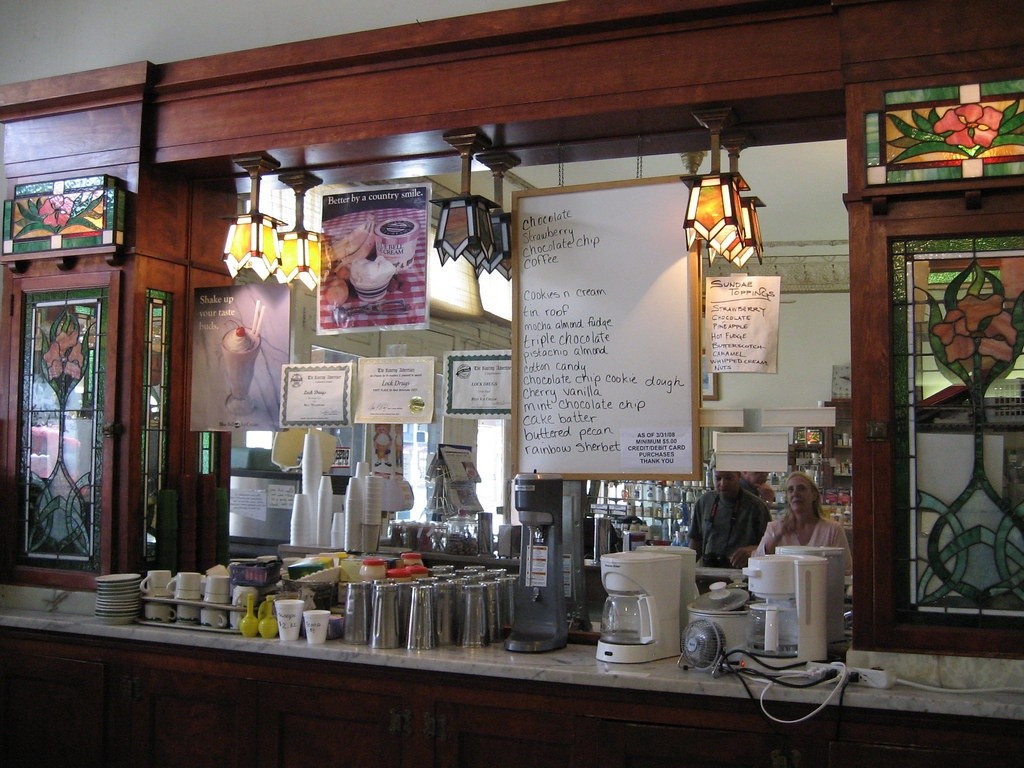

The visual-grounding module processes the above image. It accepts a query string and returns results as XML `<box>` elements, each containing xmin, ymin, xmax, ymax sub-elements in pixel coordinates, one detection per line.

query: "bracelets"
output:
<box><xmin>772</xmin><ymin>531</ymin><xmax>779</xmax><ymax>542</ymax></box>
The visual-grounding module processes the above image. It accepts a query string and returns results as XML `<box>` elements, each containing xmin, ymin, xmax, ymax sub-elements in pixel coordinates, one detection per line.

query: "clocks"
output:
<box><xmin>832</xmin><ymin>365</ymin><xmax>851</xmax><ymax>399</ymax></box>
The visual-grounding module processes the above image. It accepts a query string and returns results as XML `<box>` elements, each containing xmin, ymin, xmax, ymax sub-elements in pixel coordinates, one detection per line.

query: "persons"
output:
<box><xmin>751</xmin><ymin>471</ymin><xmax>853</xmax><ymax>575</ymax></box>
<box><xmin>686</xmin><ymin>451</ymin><xmax>774</xmax><ymax>585</ymax></box>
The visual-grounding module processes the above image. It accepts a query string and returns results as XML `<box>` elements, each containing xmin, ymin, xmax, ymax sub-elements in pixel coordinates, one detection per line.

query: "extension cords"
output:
<box><xmin>807</xmin><ymin>659</ymin><xmax>897</xmax><ymax>690</ymax></box>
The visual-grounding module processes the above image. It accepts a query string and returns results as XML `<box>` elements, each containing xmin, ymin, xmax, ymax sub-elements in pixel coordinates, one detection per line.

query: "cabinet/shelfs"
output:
<box><xmin>0</xmin><ymin>628</ymin><xmax>1024</xmax><ymax>768</ymax></box>
<box><xmin>824</xmin><ymin>401</ymin><xmax>853</xmax><ymax>492</ymax></box>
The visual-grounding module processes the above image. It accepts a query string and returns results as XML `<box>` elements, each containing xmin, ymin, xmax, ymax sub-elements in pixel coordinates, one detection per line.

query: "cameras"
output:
<box><xmin>704</xmin><ymin>553</ymin><xmax>730</xmax><ymax>568</ymax></box>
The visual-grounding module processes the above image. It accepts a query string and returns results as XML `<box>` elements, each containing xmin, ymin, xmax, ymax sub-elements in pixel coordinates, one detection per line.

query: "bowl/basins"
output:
<box><xmin>348</xmin><ymin>276</ymin><xmax>392</xmax><ymax>302</ymax></box>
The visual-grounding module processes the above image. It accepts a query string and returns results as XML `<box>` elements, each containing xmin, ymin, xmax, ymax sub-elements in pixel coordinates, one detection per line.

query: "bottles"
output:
<box><xmin>344</xmin><ymin>565</ymin><xmax>520</xmax><ymax>651</ymax></box>
<box><xmin>618</xmin><ymin>480</ymin><xmax>704</xmax><ymax>546</ymax></box>
<box><xmin>359</xmin><ymin>552</ymin><xmax>428</xmax><ymax>582</ymax></box>
<box><xmin>240</xmin><ymin>595</ymin><xmax>259</xmax><ymax>637</ymax></box>
<box><xmin>843</xmin><ymin>432</ymin><xmax>848</xmax><ymax>446</ymax></box>
<box><xmin>259</xmin><ymin>597</ymin><xmax>278</xmax><ymax>638</ymax></box>
<box><xmin>478</xmin><ymin>511</ymin><xmax>492</xmax><ymax>554</ymax></box>
<box><xmin>830</xmin><ymin>506</ymin><xmax>850</xmax><ymax>525</ymax></box>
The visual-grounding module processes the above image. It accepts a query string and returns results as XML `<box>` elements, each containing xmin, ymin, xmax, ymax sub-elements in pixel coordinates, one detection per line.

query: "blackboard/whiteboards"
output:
<box><xmin>510</xmin><ymin>174</ymin><xmax>702</xmax><ymax>482</ymax></box>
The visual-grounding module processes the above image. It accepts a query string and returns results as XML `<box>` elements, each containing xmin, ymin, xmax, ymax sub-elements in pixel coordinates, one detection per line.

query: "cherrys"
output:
<box><xmin>223</xmin><ymin>319</ymin><xmax>245</xmax><ymax>337</ymax></box>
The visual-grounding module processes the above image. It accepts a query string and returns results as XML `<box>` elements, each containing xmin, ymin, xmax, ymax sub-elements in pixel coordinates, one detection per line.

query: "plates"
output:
<box><xmin>94</xmin><ymin>572</ymin><xmax>142</xmax><ymax>624</ymax></box>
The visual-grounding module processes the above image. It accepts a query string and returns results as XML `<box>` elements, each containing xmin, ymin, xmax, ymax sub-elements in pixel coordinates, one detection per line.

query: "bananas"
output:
<box><xmin>324</xmin><ymin>214</ymin><xmax>376</xmax><ymax>274</ymax></box>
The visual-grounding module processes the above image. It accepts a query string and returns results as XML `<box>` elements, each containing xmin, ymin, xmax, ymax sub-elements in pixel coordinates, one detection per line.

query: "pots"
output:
<box><xmin>686</xmin><ymin>582</ymin><xmax>750</xmax><ymax>663</ymax></box>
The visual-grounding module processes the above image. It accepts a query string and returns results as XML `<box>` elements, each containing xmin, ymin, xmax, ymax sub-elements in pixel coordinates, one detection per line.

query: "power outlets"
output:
<box><xmin>807</xmin><ymin>663</ymin><xmax>898</xmax><ymax>690</ymax></box>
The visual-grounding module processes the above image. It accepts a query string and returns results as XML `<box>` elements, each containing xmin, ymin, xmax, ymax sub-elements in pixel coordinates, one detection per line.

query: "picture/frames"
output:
<box><xmin>701</xmin><ymin>304</ymin><xmax>719</xmax><ymax>402</ymax></box>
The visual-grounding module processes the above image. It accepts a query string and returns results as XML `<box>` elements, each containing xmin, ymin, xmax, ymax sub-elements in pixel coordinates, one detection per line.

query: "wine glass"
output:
<box><xmin>221</xmin><ymin>327</ymin><xmax>261</xmax><ymax>415</ymax></box>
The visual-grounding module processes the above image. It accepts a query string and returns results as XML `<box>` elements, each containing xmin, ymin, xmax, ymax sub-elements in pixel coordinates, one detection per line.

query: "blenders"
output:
<box><xmin>504</xmin><ymin>474</ymin><xmax>567</xmax><ymax>652</ymax></box>
<box><xmin>742</xmin><ymin>554</ymin><xmax>827</xmax><ymax>676</ymax></box>
<box><xmin>595</xmin><ymin>546</ymin><xmax>698</xmax><ymax>663</ymax></box>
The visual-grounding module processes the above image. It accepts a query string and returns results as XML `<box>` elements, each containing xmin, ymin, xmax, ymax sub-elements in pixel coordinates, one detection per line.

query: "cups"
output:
<box><xmin>177</xmin><ymin>604</ymin><xmax>201</xmax><ymax>625</ymax></box>
<box><xmin>201</xmin><ymin>575</ymin><xmax>230</xmax><ymax>603</ymax></box>
<box><xmin>290</xmin><ymin>433</ymin><xmax>383</xmax><ymax>552</ymax></box>
<box><xmin>274</xmin><ymin>600</ymin><xmax>305</xmax><ymax>640</ymax></box>
<box><xmin>140</xmin><ymin>569</ymin><xmax>171</xmax><ymax>597</ymax></box>
<box><xmin>232</xmin><ymin>586</ymin><xmax>257</xmax><ymax>606</ymax></box>
<box><xmin>302</xmin><ymin>610</ymin><xmax>330</xmax><ymax>644</ymax></box>
<box><xmin>230</xmin><ymin>611</ymin><xmax>246</xmax><ymax>629</ymax></box>
<box><xmin>145</xmin><ymin>605</ymin><xmax>176</xmax><ymax>623</ymax></box>
<box><xmin>166</xmin><ymin>572</ymin><xmax>201</xmax><ymax>600</ymax></box>
<box><xmin>201</xmin><ymin>608</ymin><xmax>227</xmax><ymax>628</ymax></box>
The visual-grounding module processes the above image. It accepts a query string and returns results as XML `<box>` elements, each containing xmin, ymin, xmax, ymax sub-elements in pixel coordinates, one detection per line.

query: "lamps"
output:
<box><xmin>679</xmin><ymin>105</ymin><xmax>766</xmax><ymax>269</ymax></box>
<box><xmin>427</xmin><ymin>131</ymin><xmax>521</xmax><ymax>283</ymax></box>
<box><xmin>221</xmin><ymin>150</ymin><xmax>323</xmax><ymax>291</ymax></box>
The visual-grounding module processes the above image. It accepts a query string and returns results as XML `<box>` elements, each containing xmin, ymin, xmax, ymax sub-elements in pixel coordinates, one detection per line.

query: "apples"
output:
<box><xmin>325</xmin><ymin>263</ymin><xmax>353</xmax><ymax>307</ymax></box>
